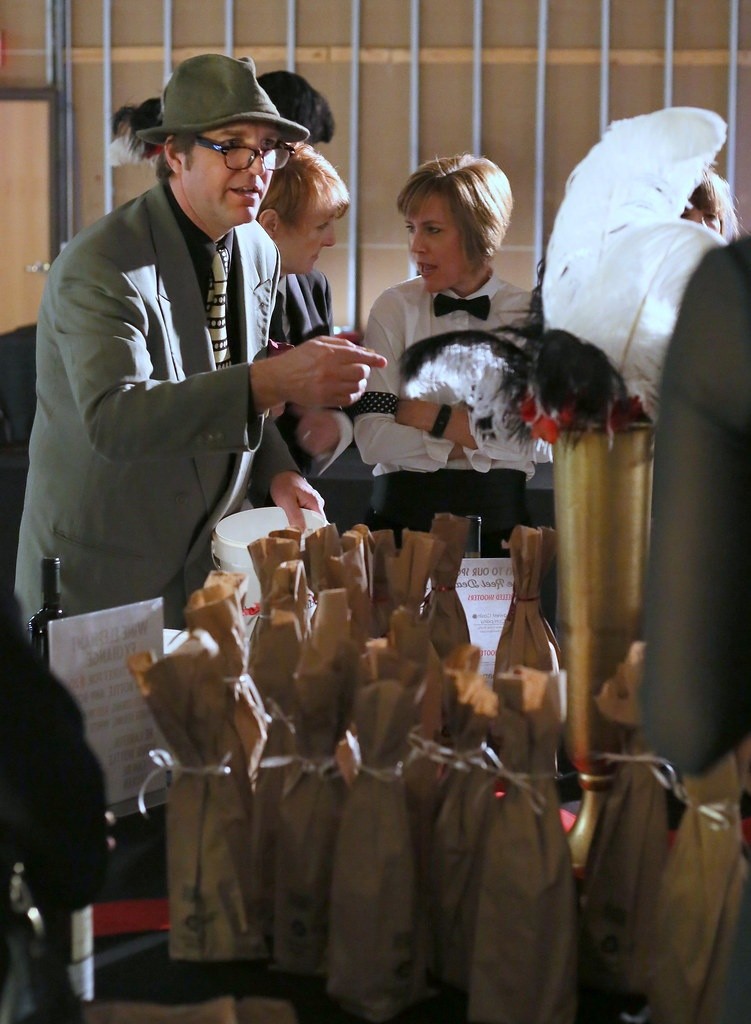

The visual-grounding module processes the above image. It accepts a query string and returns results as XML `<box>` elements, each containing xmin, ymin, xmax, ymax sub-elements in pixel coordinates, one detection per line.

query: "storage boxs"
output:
<box><xmin>69</xmin><ymin>898</ymin><xmax>172</xmax><ymax>962</ymax></box>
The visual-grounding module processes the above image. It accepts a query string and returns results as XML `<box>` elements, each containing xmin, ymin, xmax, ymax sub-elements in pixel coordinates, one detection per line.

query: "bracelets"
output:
<box><xmin>432</xmin><ymin>405</ymin><xmax>453</xmax><ymax>436</ymax></box>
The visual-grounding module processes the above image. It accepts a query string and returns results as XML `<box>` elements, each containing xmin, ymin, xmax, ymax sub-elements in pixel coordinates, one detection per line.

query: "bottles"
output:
<box><xmin>26</xmin><ymin>555</ymin><xmax>67</xmax><ymax>671</ymax></box>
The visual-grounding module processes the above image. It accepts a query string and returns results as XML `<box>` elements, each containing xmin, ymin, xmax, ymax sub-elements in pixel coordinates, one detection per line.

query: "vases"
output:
<box><xmin>548</xmin><ymin>422</ymin><xmax>658</xmax><ymax>881</ymax></box>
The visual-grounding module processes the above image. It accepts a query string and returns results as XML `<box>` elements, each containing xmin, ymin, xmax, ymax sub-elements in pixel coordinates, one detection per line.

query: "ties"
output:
<box><xmin>206</xmin><ymin>247</ymin><xmax>231</xmax><ymax>370</ymax></box>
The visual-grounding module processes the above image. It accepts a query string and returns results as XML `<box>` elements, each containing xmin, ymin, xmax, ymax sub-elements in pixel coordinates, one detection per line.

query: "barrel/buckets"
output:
<box><xmin>211</xmin><ymin>503</ymin><xmax>336</xmax><ymax>639</ymax></box>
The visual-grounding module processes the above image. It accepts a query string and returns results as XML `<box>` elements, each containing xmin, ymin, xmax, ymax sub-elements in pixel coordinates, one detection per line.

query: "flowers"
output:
<box><xmin>515</xmin><ymin>385</ymin><xmax>646</xmax><ymax>450</ymax></box>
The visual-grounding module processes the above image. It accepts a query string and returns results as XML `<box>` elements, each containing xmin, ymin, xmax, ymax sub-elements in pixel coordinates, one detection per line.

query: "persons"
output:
<box><xmin>13</xmin><ymin>54</ymin><xmax>741</xmax><ymax>660</ymax></box>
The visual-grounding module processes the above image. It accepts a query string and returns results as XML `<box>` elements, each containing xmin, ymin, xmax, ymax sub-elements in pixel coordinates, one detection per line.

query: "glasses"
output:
<box><xmin>191</xmin><ymin>135</ymin><xmax>296</xmax><ymax>172</ymax></box>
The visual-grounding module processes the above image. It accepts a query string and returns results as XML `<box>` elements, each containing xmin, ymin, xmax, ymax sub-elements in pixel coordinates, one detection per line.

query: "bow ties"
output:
<box><xmin>433</xmin><ymin>294</ymin><xmax>491</xmax><ymax>321</ymax></box>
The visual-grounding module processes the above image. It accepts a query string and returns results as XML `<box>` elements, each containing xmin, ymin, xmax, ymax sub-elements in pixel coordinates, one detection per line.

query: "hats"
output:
<box><xmin>136</xmin><ymin>54</ymin><xmax>309</xmax><ymax>149</ymax></box>
